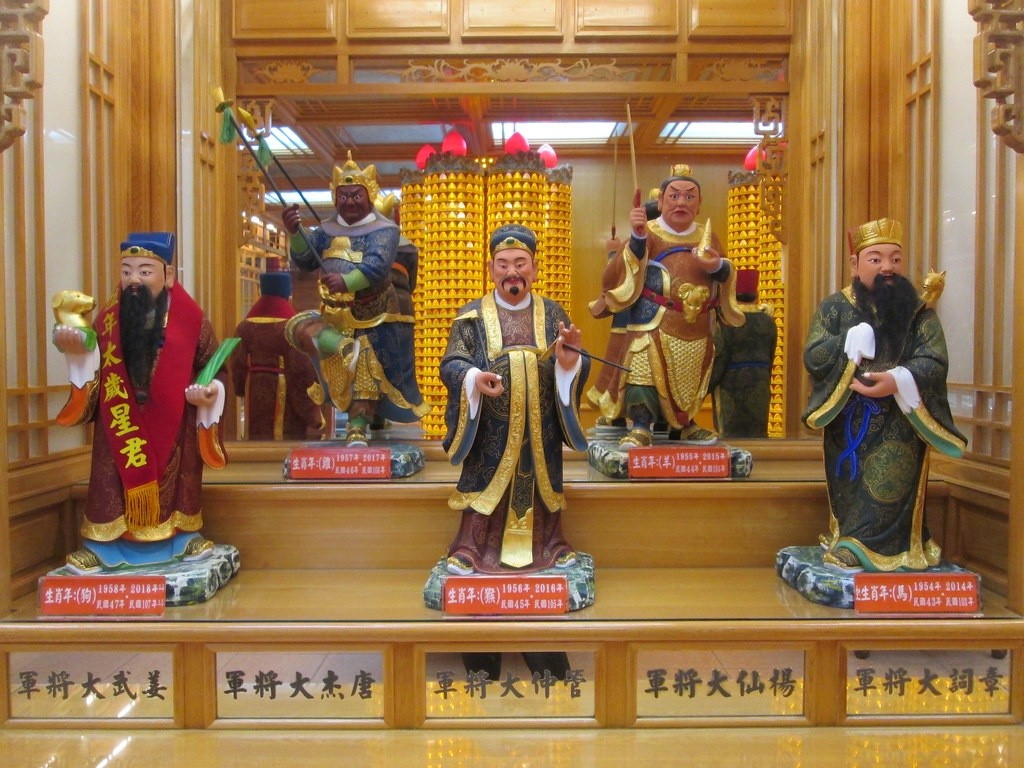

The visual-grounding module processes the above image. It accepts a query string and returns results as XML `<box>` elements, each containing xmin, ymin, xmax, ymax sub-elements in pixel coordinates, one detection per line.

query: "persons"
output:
<box><xmin>437</xmin><ymin>226</ymin><xmax>592</xmax><ymax>574</ymax></box>
<box><xmin>703</xmin><ymin>268</ymin><xmax>778</xmax><ymax>438</ymax></box>
<box><xmin>53</xmin><ymin>232</ymin><xmax>231</xmax><ymax>574</ymax></box>
<box><xmin>359</xmin><ymin>163</ymin><xmax>433</xmax><ymax>435</ymax></box>
<box><xmin>586</xmin><ymin>185</ymin><xmax>662</xmax><ymax>436</ymax></box>
<box><xmin>597</xmin><ymin>163</ymin><xmax>746</xmax><ymax>451</ymax></box>
<box><xmin>800</xmin><ymin>216</ymin><xmax>968</xmax><ymax>572</ymax></box>
<box><xmin>230</xmin><ymin>273</ymin><xmax>327</xmax><ymax>441</ymax></box>
<box><xmin>282</xmin><ymin>149</ymin><xmax>423</xmax><ymax>447</ymax></box>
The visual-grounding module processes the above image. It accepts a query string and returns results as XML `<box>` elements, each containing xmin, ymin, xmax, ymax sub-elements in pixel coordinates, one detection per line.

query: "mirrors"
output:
<box><xmin>226</xmin><ymin>38</ymin><xmax>848</xmax><ymax>441</ymax></box>
